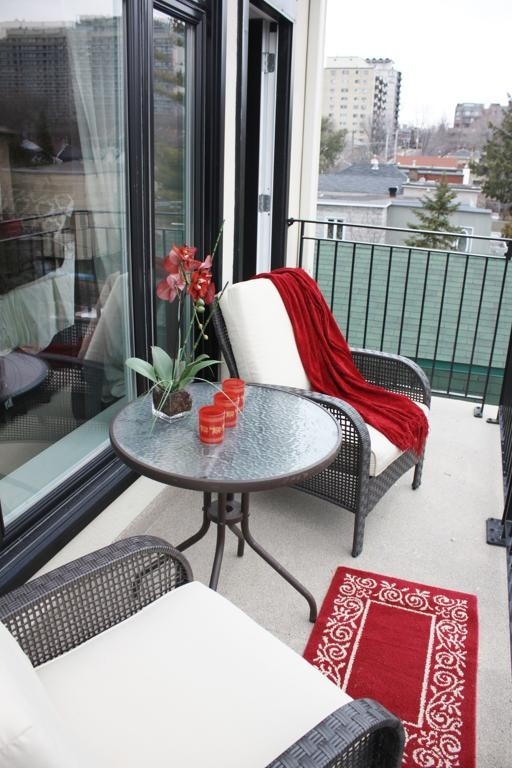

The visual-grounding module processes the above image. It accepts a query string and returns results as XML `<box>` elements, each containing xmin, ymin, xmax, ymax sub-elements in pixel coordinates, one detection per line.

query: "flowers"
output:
<box><xmin>124</xmin><ymin>240</ymin><xmax>229</xmax><ymax>389</ymax></box>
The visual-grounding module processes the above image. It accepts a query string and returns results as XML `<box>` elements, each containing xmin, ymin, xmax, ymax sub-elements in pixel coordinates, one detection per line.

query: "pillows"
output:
<box><xmin>3</xmin><ymin>188</ymin><xmax>75</xmax><ymax>239</ymax></box>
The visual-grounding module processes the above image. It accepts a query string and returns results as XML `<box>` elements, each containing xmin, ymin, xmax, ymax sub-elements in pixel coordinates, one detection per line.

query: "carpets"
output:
<box><xmin>302</xmin><ymin>566</ymin><xmax>480</xmax><ymax>768</ymax></box>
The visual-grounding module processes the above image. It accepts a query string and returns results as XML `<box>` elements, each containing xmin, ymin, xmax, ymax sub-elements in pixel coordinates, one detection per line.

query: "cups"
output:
<box><xmin>198</xmin><ymin>406</ymin><xmax>225</xmax><ymax>444</ymax></box>
<box><xmin>222</xmin><ymin>379</ymin><xmax>245</xmax><ymax>412</ymax></box>
<box><xmin>213</xmin><ymin>392</ymin><xmax>238</xmax><ymax>426</ymax></box>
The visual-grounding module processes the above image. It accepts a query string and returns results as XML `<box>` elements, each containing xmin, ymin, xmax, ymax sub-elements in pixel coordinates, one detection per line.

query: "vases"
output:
<box><xmin>150</xmin><ymin>387</ymin><xmax>195</xmax><ymax>424</ymax></box>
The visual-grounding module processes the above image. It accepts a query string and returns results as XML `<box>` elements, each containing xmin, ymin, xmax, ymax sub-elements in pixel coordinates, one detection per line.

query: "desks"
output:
<box><xmin>109</xmin><ymin>381</ymin><xmax>343</xmax><ymax>623</ymax></box>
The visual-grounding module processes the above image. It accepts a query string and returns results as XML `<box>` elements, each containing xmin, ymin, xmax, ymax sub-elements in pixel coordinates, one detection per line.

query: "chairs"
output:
<box><xmin>211</xmin><ymin>268</ymin><xmax>431</xmax><ymax>558</ymax></box>
<box><xmin>0</xmin><ymin>534</ymin><xmax>408</xmax><ymax>767</ymax></box>
